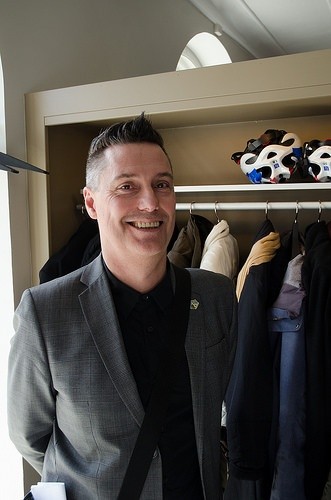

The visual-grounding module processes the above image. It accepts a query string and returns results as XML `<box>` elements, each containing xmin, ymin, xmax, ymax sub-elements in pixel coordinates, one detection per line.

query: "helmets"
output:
<box><xmin>231</xmin><ymin>129</ymin><xmax>302</xmax><ymax>183</ymax></box>
<box><xmin>297</xmin><ymin>139</ymin><xmax>331</xmax><ymax>183</ymax></box>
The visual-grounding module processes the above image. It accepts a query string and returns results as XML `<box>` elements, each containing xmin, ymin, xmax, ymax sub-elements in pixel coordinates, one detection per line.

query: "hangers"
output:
<box><xmin>184</xmin><ymin>200</ymin><xmax>325</xmax><ymax>265</ymax></box>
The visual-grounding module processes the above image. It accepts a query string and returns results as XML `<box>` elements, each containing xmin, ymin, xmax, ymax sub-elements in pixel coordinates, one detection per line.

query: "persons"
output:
<box><xmin>6</xmin><ymin>111</ymin><xmax>241</xmax><ymax>500</ymax></box>
